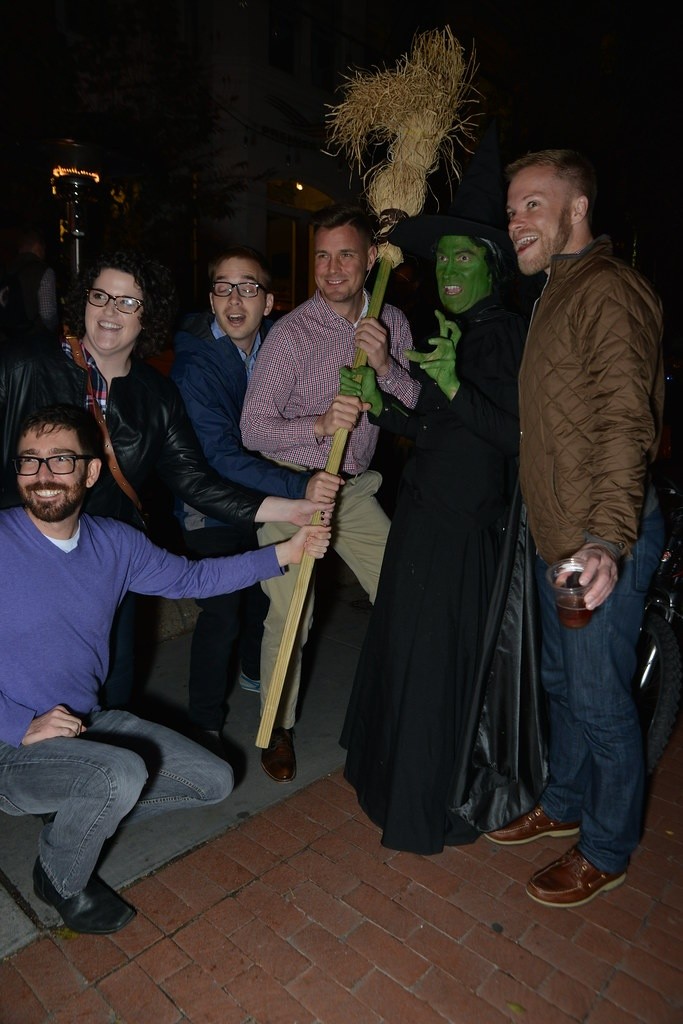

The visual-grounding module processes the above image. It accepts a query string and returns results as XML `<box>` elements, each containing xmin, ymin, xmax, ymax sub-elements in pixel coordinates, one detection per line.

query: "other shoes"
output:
<box><xmin>239</xmin><ymin>672</ymin><xmax>263</xmax><ymax>693</ymax></box>
<box><xmin>195</xmin><ymin>730</ymin><xmax>220</xmax><ymax>754</ymax></box>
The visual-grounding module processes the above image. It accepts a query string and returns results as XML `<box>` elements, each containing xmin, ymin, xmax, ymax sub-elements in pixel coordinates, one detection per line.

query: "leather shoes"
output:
<box><xmin>484</xmin><ymin>806</ymin><xmax>582</xmax><ymax>845</ymax></box>
<box><xmin>260</xmin><ymin>726</ymin><xmax>296</xmax><ymax>783</ymax></box>
<box><xmin>526</xmin><ymin>845</ymin><xmax>627</xmax><ymax>908</ymax></box>
<box><xmin>31</xmin><ymin>813</ymin><xmax>59</xmax><ymax>826</ymax></box>
<box><xmin>32</xmin><ymin>856</ymin><xmax>135</xmax><ymax>934</ymax></box>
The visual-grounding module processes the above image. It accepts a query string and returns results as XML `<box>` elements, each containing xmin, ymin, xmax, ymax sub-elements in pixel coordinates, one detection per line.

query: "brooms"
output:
<box><xmin>254</xmin><ymin>19</ymin><xmax>485</xmax><ymax>753</ymax></box>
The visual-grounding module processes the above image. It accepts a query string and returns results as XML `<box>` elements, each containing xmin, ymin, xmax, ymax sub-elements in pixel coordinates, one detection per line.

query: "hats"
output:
<box><xmin>399</xmin><ymin>184</ymin><xmax>517</xmax><ymax>259</ymax></box>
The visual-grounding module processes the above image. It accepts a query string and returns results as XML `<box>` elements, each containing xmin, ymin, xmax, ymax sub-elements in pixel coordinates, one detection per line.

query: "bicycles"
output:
<box><xmin>630</xmin><ymin>455</ymin><xmax>683</xmax><ymax>777</ymax></box>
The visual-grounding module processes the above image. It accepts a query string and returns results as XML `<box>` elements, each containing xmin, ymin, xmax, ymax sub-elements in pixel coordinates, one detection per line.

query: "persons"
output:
<box><xmin>240</xmin><ymin>202</ymin><xmax>422</xmax><ymax>784</ymax></box>
<box><xmin>0</xmin><ymin>403</ymin><xmax>332</xmax><ymax>936</ymax></box>
<box><xmin>338</xmin><ymin>161</ymin><xmax>550</xmax><ymax>856</ymax></box>
<box><xmin>0</xmin><ymin>222</ymin><xmax>59</xmax><ymax>340</ymax></box>
<box><xmin>0</xmin><ymin>244</ymin><xmax>336</xmax><ymax>712</ymax></box>
<box><xmin>169</xmin><ymin>245</ymin><xmax>345</xmax><ymax>777</ymax></box>
<box><xmin>483</xmin><ymin>151</ymin><xmax>665</xmax><ymax>908</ymax></box>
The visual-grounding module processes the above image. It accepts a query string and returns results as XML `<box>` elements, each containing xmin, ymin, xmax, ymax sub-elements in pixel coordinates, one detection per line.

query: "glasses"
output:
<box><xmin>84</xmin><ymin>288</ymin><xmax>144</xmax><ymax>315</ymax></box>
<box><xmin>10</xmin><ymin>455</ymin><xmax>96</xmax><ymax>476</ymax></box>
<box><xmin>209</xmin><ymin>281</ymin><xmax>268</xmax><ymax>298</ymax></box>
